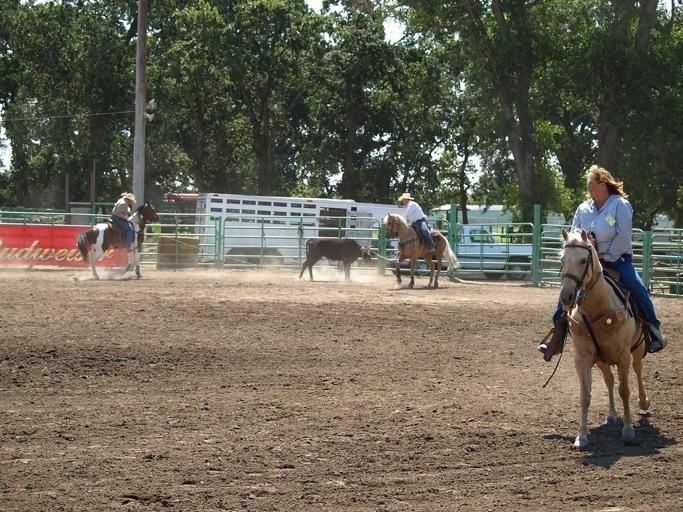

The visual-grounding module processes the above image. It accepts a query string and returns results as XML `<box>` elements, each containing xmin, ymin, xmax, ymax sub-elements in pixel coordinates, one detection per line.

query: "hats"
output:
<box><xmin>399</xmin><ymin>193</ymin><xmax>414</xmax><ymax>201</ymax></box>
<box><xmin>123</xmin><ymin>193</ymin><xmax>136</xmax><ymax>203</ymax></box>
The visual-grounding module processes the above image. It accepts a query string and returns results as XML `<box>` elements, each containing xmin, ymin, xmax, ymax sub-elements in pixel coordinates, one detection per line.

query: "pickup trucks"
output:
<box><xmin>394</xmin><ymin>224</ymin><xmax>535</xmax><ymax>278</ymax></box>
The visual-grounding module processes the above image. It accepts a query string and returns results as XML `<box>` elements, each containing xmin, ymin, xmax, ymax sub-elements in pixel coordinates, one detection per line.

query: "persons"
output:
<box><xmin>398</xmin><ymin>193</ymin><xmax>436</xmax><ymax>251</ymax></box>
<box><xmin>536</xmin><ymin>163</ymin><xmax>667</xmax><ymax>353</ymax></box>
<box><xmin>112</xmin><ymin>192</ymin><xmax>136</xmax><ymax>251</ymax></box>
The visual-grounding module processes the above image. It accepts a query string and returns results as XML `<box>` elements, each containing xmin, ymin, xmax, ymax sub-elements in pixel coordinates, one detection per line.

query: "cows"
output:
<box><xmin>299</xmin><ymin>237</ymin><xmax>374</xmax><ymax>282</ymax></box>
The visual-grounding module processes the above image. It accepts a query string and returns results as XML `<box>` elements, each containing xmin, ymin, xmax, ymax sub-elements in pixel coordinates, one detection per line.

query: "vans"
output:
<box><xmin>431</xmin><ymin>203</ymin><xmax>679</xmax><ymax>259</ymax></box>
<box><xmin>194</xmin><ymin>191</ymin><xmax>426</xmax><ymax>268</ymax></box>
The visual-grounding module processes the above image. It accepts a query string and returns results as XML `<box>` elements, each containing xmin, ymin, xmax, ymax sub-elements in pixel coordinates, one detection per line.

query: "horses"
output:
<box><xmin>557</xmin><ymin>226</ymin><xmax>652</xmax><ymax>452</ymax></box>
<box><xmin>383</xmin><ymin>212</ymin><xmax>460</xmax><ymax>288</ymax></box>
<box><xmin>75</xmin><ymin>199</ymin><xmax>160</xmax><ymax>281</ymax></box>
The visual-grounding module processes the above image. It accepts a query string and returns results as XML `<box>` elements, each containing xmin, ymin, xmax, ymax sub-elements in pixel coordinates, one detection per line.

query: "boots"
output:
<box><xmin>538</xmin><ymin>320</ymin><xmax>569</xmax><ymax>354</ymax></box>
<box><xmin>648</xmin><ymin>320</ymin><xmax>662</xmax><ymax>352</ymax></box>
